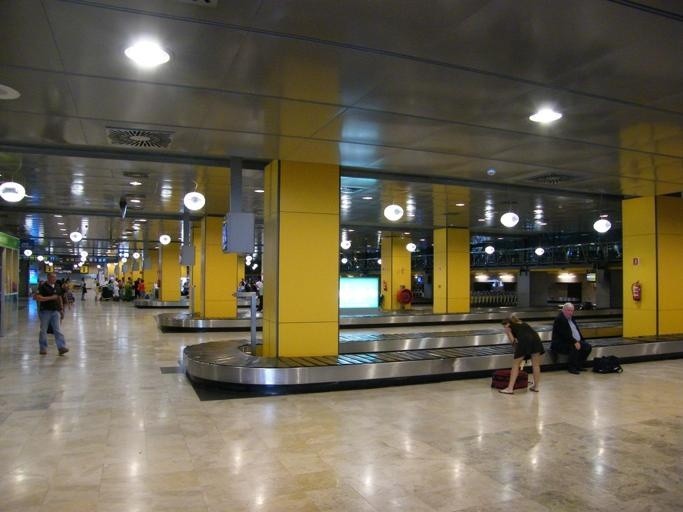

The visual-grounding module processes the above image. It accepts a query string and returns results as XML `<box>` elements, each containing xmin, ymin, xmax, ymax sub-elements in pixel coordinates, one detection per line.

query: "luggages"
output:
<box><xmin>491</xmin><ymin>368</ymin><xmax>529</xmax><ymax>389</ymax></box>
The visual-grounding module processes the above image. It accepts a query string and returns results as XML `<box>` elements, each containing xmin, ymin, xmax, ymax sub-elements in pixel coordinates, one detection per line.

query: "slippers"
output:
<box><xmin>497</xmin><ymin>388</ymin><xmax>515</xmax><ymax>395</ymax></box>
<box><xmin>529</xmin><ymin>386</ymin><xmax>540</xmax><ymax>392</ymax></box>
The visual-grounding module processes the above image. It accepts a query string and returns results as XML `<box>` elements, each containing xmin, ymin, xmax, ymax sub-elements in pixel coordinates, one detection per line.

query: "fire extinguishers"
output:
<box><xmin>157</xmin><ymin>280</ymin><xmax>160</xmax><ymax>287</ymax></box>
<box><xmin>383</xmin><ymin>281</ymin><xmax>386</xmax><ymax>290</ymax></box>
<box><xmin>631</xmin><ymin>281</ymin><xmax>640</xmax><ymax>301</ymax></box>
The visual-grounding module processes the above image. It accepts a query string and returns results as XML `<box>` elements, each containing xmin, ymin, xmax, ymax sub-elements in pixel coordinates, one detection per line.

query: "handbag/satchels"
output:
<box><xmin>592</xmin><ymin>355</ymin><xmax>623</xmax><ymax>374</ymax></box>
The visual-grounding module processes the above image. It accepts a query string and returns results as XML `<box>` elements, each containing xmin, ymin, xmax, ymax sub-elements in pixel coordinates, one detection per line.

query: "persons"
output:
<box><xmin>35</xmin><ymin>280</ymin><xmax>44</xmax><ymax>315</ymax></box>
<box><xmin>548</xmin><ymin>301</ymin><xmax>592</xmax><ymax>375</ymax></box>
<box><xmin>496</xmin><ymin>315</ymin><xmax>544</xmax><ymax>396</ymax></box>
<box><xmin>55</xmin><ymin>278</ymin><xmax>72</xmax><ymax>325</ymax></box>
<box><xmin>238</xmin><ymin>277</ymin><xmax>263</xmax><ymax>312</ymax></box>
<box><xmin>80</xmin><ymin>277</ymin><xmax>86</xmax><ymax>301</ymax></box>
<box><xmin>34</xmin><ymin>270</ymin><xmax>71</xmax><ymax>355</ymax></box>
<box><xmin>183</xmin><ymin>278</ymin><xmax>189</xmax><ymax>286</ymax></box>
<box><xmin>94</xmin><ymin>276</ymin><xmax>145</xmax><ymax>303</ymax></box>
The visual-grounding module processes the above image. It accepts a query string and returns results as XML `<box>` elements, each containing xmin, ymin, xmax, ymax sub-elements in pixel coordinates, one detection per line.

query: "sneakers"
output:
<box><xmin>39</xmin><ymin>349</ymin><xmax>48</xmax><ymax>355</ymax></box>
<box><xmin>58</xmin><ymin>347</ymin><xmax>69</xmax><ymax>356</ymax></box>
<box><xmin>567</xmin><ymin>367</ymin><xmax>579</xmax><ymax>375</ymax></box>
<box><xmin>578</xmin><ymin>366</ymin><xmax>589</xmax><ymax>372</ymax></box>
